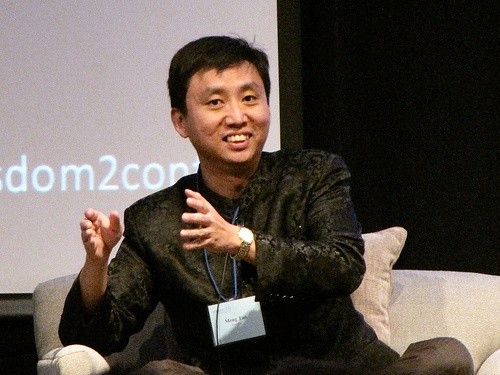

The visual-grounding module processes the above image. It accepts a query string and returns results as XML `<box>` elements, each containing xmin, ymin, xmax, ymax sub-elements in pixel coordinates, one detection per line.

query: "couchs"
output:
<box><xmin>33</xmin><ymin>269</ymin><xmax>500</xmax><ymax>375</ymax></box>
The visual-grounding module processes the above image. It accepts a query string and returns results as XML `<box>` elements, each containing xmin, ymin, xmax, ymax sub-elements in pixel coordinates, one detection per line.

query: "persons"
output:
<box><xmin>57</xmin><ymin>36</ymin><xmax>473</xmax><ymax>375</ymax></box>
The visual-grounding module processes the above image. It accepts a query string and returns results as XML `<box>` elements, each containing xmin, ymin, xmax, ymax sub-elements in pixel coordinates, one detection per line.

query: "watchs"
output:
<box><xmin>228</xmin><ymin>224</ymin><xmax>254</xmax><ymax>262</ymax></box>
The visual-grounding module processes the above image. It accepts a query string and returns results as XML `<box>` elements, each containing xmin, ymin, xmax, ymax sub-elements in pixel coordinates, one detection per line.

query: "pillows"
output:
<box><xmin>351</xmin><ymin>226</ymin><xmax>407</xmax><ymax>346</ymax></box>
